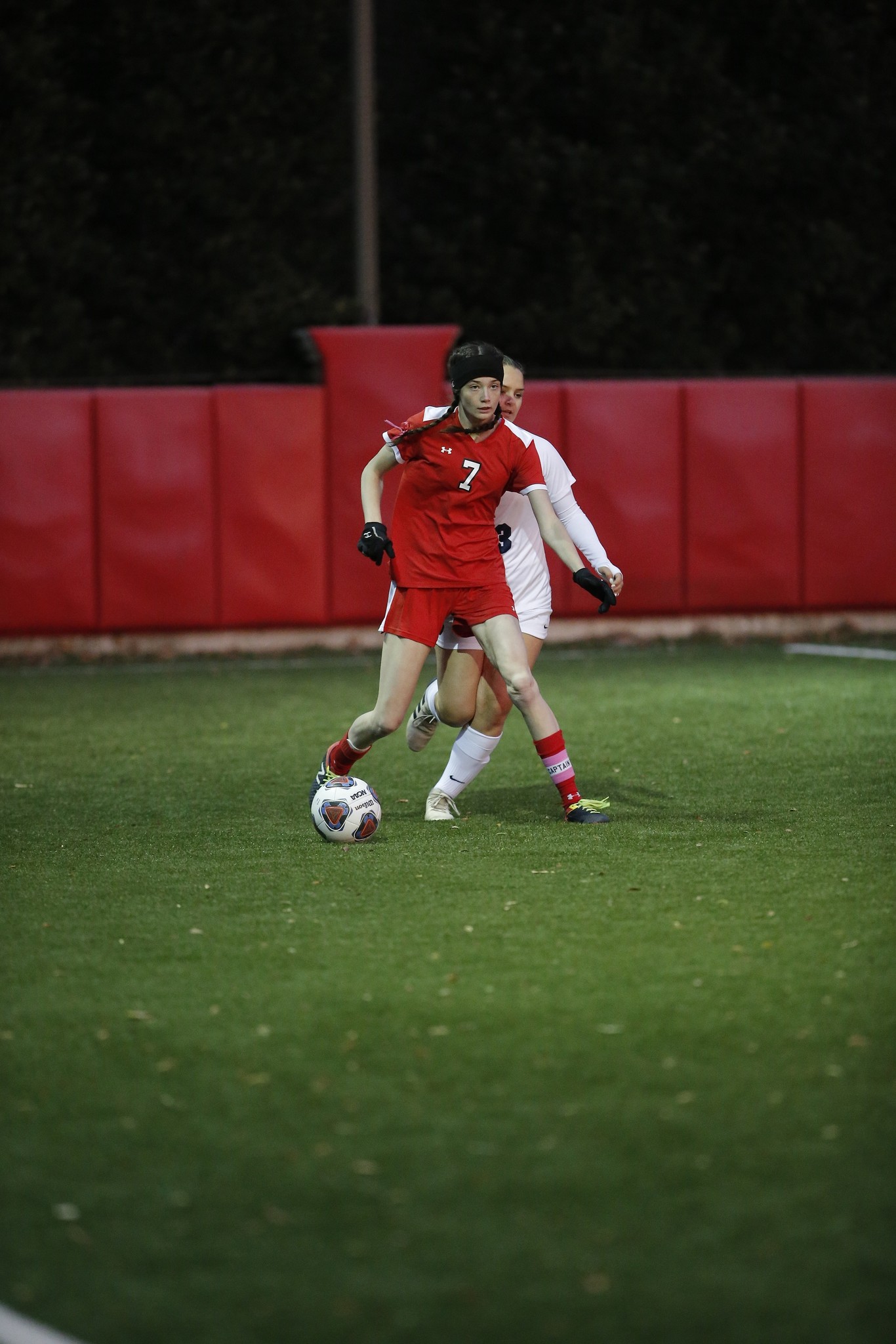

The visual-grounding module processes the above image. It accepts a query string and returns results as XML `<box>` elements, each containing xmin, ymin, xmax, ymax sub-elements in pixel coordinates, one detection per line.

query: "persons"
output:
<box><xmin>308</xmin><ymin>339</ymin><xmax>619</xmax><ymax>824</ymax></box>
<box><xmin>405</xmin><ymin>351</ymin><xmax>628</xmax><ymax>823</ymax></box>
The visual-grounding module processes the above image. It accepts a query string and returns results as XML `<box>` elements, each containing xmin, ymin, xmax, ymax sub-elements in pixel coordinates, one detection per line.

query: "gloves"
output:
<box><xmin>359</xmin><ymin>522</ymin><xmax>394</xmax><ymax>566</ymax></box>
<box><xmin>570</xmin><ymin>568</ymin><xmax>617</xmax><ymax>615</ymax></box>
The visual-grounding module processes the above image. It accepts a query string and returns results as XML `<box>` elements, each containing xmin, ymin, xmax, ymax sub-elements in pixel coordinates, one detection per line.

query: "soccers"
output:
<box><xmin>312</xmin><ymin>776</ymin><xmax>380</xmax><ymax>842</ymax></box>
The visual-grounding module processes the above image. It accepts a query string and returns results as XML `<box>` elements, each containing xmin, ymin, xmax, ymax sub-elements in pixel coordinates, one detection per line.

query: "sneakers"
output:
<box><xmin>564</xmin><ymin>804</ymin><xmax>610</xmax><ymax>823</ymax></box>
<box><xmin>407</xmin><ymin>679</ymin><xmax>438</xmax><ymax>753</ymax></box>
<box><xmin>309</xmin><ymin>740</ymin><xmax>350</xmax><ymax>808</ymax></box>
<box><xmin>426</xmin><ymin>791</ymin><xmax>454</xmax><ymax>821</ymax></box>
